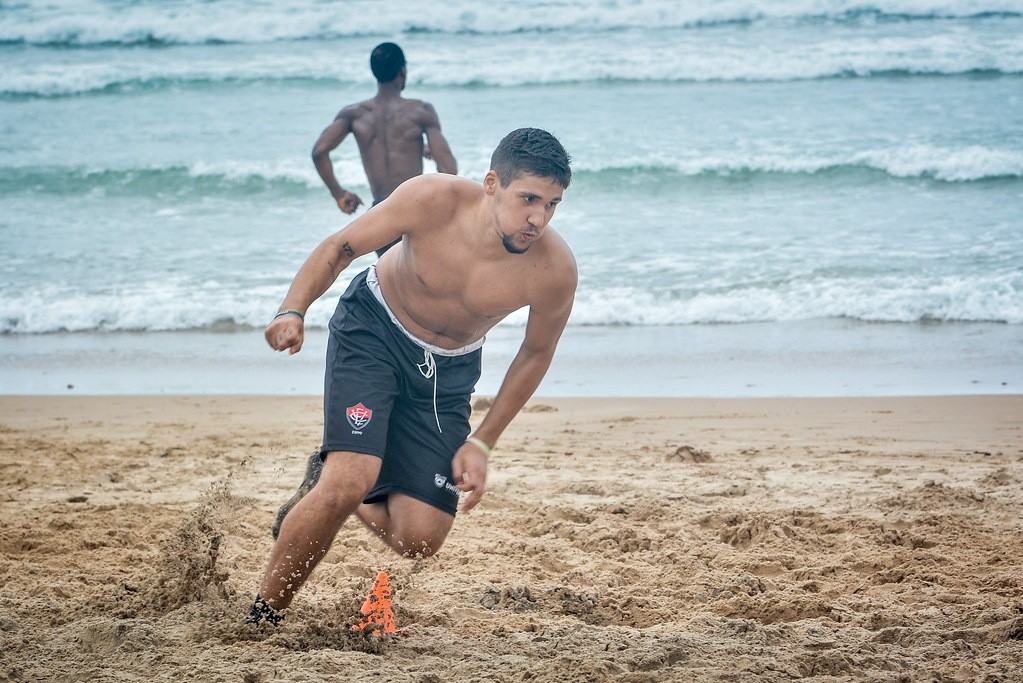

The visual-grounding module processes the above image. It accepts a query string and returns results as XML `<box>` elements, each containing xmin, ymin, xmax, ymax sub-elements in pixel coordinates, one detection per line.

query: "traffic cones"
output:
<box><xmin>349</xmin><ymin>569</ymin><xmax>402</xmax><ymax>641</ymax></box>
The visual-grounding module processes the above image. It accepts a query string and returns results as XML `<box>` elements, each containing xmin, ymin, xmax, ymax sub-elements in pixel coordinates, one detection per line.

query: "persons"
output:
<box><xmin>244</xmin><ymin>128</ymin><xmax>577</xmax><ymax>627</ymax></box>
<box><xmin>310</xmin><ymin>42</ymin><xmax>457</xmax><ymax>257</ymax></box>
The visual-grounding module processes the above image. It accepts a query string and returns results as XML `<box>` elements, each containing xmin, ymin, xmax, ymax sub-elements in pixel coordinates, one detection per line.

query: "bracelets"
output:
<box><xmin>274</xmin><ymin>308</ymin><xmax>303</xmax><ymax>319</ymax></box>
<box><xmin>464</xmin><ymin>436</ymin><xmax>491</xmax><ymax>456</ymax></box>
